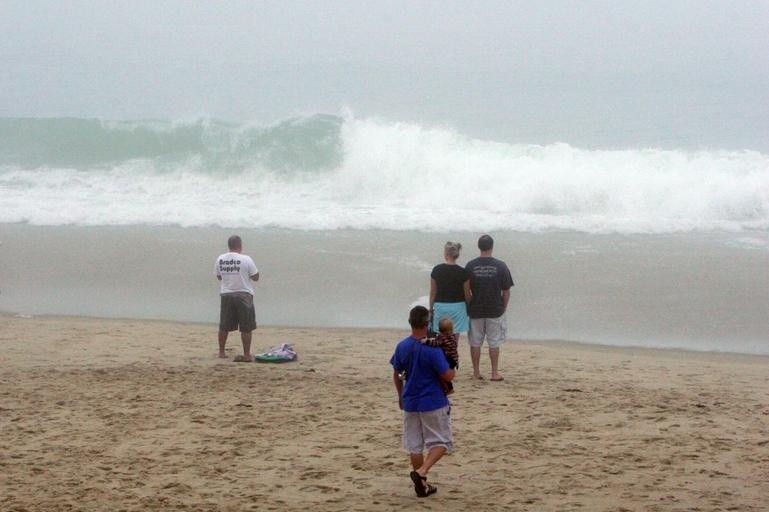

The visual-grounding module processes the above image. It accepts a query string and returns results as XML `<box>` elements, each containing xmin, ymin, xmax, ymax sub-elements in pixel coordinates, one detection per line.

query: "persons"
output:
<box><xmin>214</xmin><ymin>236</ymin><xmax>261</xmax><ymax>359</ymax></box>
<box><xmin>428</xmin><ymin>241</ymin><xmax>471</xmax><ymax>349</ymax></box>
<box><xmin>463</xmin><ymin>234</ymin><xmax>515</xmax><ymax>380</ymax></box>
<box><xmin>389</xmin><ymin>306</ymin><xmax>455</xmax><ymax>497</ymax></box>
<box><xmin>419</xmin><ymin>318</ymin><xmax>459</xmax><ymax>397</ymax></box>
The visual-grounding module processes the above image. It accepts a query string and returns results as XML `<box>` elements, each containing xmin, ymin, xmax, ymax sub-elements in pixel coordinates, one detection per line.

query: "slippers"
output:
<box><xmin>233</xmin><ymin>355</ymin><xmax>252</xmax><ymax>362</ymax></box>
<box><xmin>490</xmin><ymin>375</ymin><xmax>504</xmax><ymax>381</ymax></box>
<box><xmin>410</xmin><ymin>471</ymin><xmax>428</xmax><ymax>496</ymax></box>
<box><xmin>471</xmin><ymin>375</ymin><xmax>485</xmax><ymax>380</ymax></box>
<box><xmin>417</xmin><ymin>485</ymin><xmax>438</xmax><ymax>497</ymax></box>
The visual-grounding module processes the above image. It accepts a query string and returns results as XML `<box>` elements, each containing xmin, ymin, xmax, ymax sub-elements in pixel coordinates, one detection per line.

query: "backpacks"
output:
<box><xmin>255</xmin><ymin>344</ymin><xmax>296</xmax><ymax>363</ymax></box>
